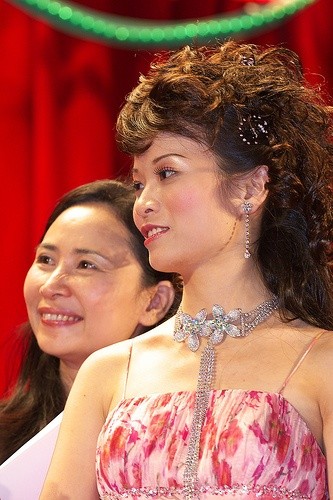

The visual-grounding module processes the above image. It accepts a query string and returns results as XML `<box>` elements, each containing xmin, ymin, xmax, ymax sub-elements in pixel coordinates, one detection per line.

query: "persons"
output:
<box><xmin>39</xmin><ymin>40</ymin><xmax>332</xmax><ymax>500</ymax></box>
<box><xmin>0</xmin><ymin>179</ymin><xmax>187</xmax><ymax>468</ymax></box>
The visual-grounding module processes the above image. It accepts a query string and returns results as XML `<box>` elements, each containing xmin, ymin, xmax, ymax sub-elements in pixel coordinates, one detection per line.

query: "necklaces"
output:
<box><xmin>172</xmin><ymin>292</ymin><xmax>284</xmax><ymax>497</ymax></box>
